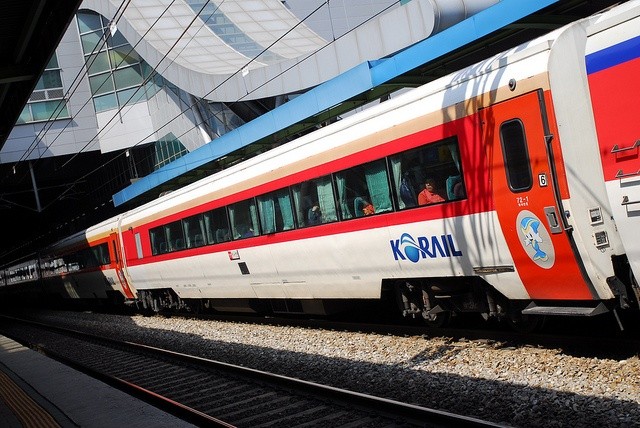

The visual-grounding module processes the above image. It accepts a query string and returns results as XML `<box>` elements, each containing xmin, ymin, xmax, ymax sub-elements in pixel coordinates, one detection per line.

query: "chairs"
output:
<box><xmin>216</xmin><ymin>227</ymin><xmax>226</xmax><ymax>242</ymax></box>
<box><xmin>175</xmin><ymin>237</ymin><xmax>184</xmax><ymax>251</ymax></box>
<box><xmin>446</xmin><ymin>175</ymin><xmax>461</xmax><ymax>199</ymax></box>
<box><xmin>308</xmin><ymin>208</ymin><xmax>319</xmax><ymax>224</ymax></box>
<box><xmin>160</xmin><ymin>243</ymin><xmax>166</xmax><ymax>253</ymax></box>
<box><xmin>355</xmin><ymin>196</ymin><xmax>367</xmax><ymax>217</ymax></box>
<box><xmin>195</xmin><ymin>234</ymin><xmax>202</xmax><ymax>246</ymax></box>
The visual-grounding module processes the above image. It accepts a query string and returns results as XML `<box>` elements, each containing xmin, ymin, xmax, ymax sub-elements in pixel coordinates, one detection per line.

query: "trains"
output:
<box><xmin>0</xmin><ymin>0</ymin><xmax>640</xmax><ymax>321</ymax></box>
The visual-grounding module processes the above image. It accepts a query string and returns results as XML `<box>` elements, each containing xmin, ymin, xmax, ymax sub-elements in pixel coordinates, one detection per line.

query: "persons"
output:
<box><xmin>418</xmin><ymin>178</ymin><xmax>448</xmax><ymax>205</ymax></box>
